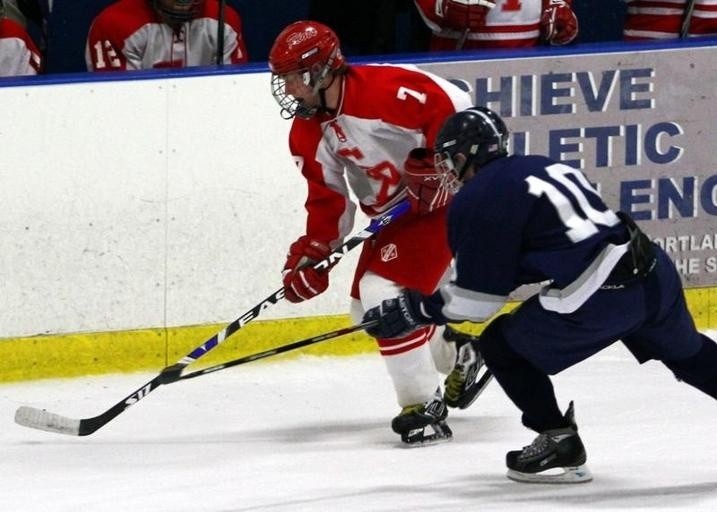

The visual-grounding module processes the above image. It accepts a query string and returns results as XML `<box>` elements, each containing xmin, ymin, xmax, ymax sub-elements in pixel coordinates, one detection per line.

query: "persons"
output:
<box><xmin>362</xmin><ymin>106</ymin><xmax>717</xmax><ymax>474</ymax></box>
<box><xmin>0</xmin><ymin>0</ymin><xmax>43</xmax><ymax>76</ymax></box>
<box><xmin>414</xmin><ymin>0</ymin><xmax>579</xmax><ymax>52</ymax></box>
<box><xmin>268</xmin><ymin>20</ymin><xmax>480</xmax><ymax>432</ymax></box>
<box><xmin>85</xmin><ymin>0</ymin><xmax>248</xmax><ymax>72</ymax></box>
<box><xmin>621</xmin><ymin>0</ymin><xmax>717</xmax><ymax>41</ymax></box>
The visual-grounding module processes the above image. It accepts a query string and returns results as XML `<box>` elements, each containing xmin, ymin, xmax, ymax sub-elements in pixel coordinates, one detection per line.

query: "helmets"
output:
<box><xmin>432</xmin><ymin>106</ymin><xmax>510</xmax><ymax>175</ymax></box>
<box><xmin>269</xmin><ymin>20</ymin><xmax>343</xmax><ymax>82</ymax></box>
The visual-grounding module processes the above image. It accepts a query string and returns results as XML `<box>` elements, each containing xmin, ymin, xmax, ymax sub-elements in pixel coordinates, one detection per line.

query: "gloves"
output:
<box><xmin>440</xmin><ymin>0</ymin><xmax>496</xmax><ymax>32</ymax></box>
<box><xmin>398</xmin><ymin>148</ymin><xmax>453</xmax><ymax>221</ymax></box>
<box><xmin>361</xmin><ymin>289</ymin><xmax>451</xmax><ymax>340</ymax></box>
<box><xmin>282</xmin><ymin>236</ymin><xmax>334</xmax><ymax>303</ymax></box>
<box><xmin>541</xmin><ymin>0</ymin><xmax>581</xmax><ymax>48</ymax></box>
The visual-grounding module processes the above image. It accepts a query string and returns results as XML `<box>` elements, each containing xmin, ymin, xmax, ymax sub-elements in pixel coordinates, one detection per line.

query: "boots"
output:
<box><xmin>444</xmin><ymin>339</ymin><xmax>481</xmax><ymax>402</ymax></box>
<box><xmin>506</xmin><ymin>398</ymin><xmax>586</xmax><ymax>473</ymax></box>
<box><xmin>391</xmin><ymin>386</ymin><xmax>448</xmax><ymax>434</ymax></box>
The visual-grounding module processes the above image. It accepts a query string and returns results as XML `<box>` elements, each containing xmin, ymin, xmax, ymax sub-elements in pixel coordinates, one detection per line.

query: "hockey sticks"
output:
<box><xmin>161</xmin><ymin>322</ymin><xmax>387</xmax><ymax>383</ymax></box>
<box><xmin>15</xmin><ymin>198</ymin><xmax>411</xmax><ymax>436</ymax></box>
